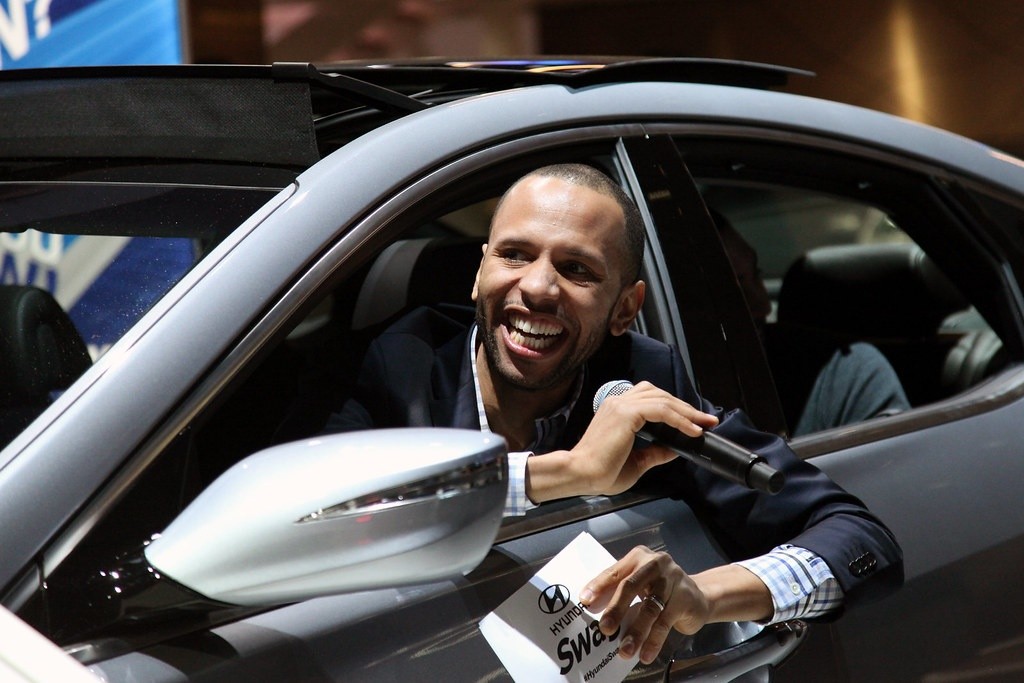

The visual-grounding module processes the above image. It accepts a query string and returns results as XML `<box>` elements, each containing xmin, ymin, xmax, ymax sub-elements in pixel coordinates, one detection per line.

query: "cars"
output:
<box><xmin>0</xmin><ymin>53</ymin><xmax>1024</xmax><ymax>683</ymax></box>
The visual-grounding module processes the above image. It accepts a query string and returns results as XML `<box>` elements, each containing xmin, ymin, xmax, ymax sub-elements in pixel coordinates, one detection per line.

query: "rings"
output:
<box><xmin>643</xmin><ymin>594</ymin><xmax>666</xmax><ymax>612</ymax></box>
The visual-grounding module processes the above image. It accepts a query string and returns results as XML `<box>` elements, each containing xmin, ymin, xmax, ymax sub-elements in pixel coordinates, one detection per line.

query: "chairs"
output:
<box><xmin>350</xmin><ymin>239</ymin><xmax>438</xmax><ymax>344</ymax></box>
<box><xmin>776</xmin><ymin>244</ymin><xmax>1002</xmax><ymax>408</ymax></box>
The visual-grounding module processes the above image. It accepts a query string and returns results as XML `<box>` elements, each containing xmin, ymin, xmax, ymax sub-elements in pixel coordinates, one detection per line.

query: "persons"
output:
<box><xmin>705</xmin><ymin>204</ymin><xmax>912</xmax><ymax>438</ymax></box>
<box><xmin>272</xmin><ymin>163</ymin><xmax>904</xmax><ymax>665</ymax></box>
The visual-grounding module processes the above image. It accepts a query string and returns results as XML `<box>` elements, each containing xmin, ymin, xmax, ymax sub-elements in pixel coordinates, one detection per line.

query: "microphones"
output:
<box><xmin>592</xmin><ymin>379</ymin><xmax>786</xmax><ymax>495</ymax></box>
